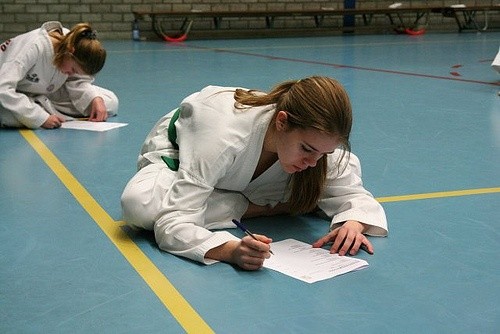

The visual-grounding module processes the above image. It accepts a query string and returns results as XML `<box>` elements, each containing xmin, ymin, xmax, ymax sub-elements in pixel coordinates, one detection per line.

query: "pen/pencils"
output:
<box><xmin>45</xmin><ymin>98</ymin><xmax>55</xmax><ymax>115</ymax></box>
<box><xmin>231</xmin><ymin>218</ymin><xmax>275</xmax><ymax>256</ymax></box>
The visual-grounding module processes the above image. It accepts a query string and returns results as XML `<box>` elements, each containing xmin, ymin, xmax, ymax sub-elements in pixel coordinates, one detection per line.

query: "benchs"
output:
<box><xmin>129</xmin><ymin>5</ymin><xmax>500</xmax><ymax>44</ymax></box>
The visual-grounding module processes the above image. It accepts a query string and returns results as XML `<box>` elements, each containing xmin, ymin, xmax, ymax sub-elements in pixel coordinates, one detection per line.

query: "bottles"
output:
<box><xmin>133</xmin><ymin>19</ymin><xmax>140</xmax><ymax>41</ymax></box>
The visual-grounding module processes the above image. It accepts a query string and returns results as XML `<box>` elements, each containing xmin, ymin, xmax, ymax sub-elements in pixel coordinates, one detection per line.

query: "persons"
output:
<box><xmin>0</xmin><ymin>21</ymin><xmax>119</xmax><ymax>129</ymax></box>
<box><xmin>121</xmin><ymin>76</ymin><xmax>389</xmax><ymax>272</ymax></box>
